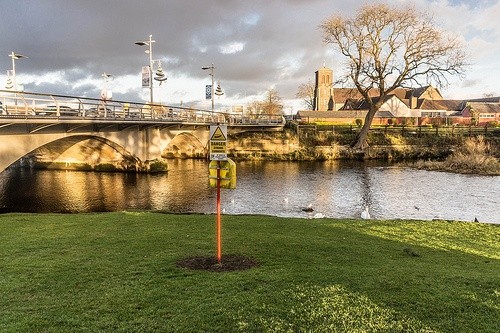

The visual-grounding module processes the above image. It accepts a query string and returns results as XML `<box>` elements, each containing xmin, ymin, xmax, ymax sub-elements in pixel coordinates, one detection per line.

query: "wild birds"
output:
<box><xmin>474</xmin><ymin>217</ymin><xmax>479</xmax><ymax>223</ymax></box>
<box><xmin>482</xmin><ymin>260</ymin><xmax>491</xmax><ymax>264</ymax></box>
<box><xmin>414</xmin><ymin>205</ymin><xmax>420</xmax><ymax>211</ymax></box>
<box><xmin>302</xmin><ymin>207</ymin><xmax>315</xmax><ymax>213</ymax></box>
<box><xmin>411</xmin><ymin>251</ymin><xmax>421</xmax><ymax>258</ymax></box>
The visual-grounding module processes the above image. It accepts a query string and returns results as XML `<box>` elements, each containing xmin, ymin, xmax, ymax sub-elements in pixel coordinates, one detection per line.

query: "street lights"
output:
<box><xmin>203</xmin><ymin>63</ymin><xmax>216</xmax><ymax>111</ymax></box>
<box><xmin>9</xmin><ymin>50</ymin><xmax>26</xmax><ymax>93</ymax></box>
<box><xmin>134</xmin><ymin>33</ymin><xmax>156</xmax><ymax>106</ymax></box>
